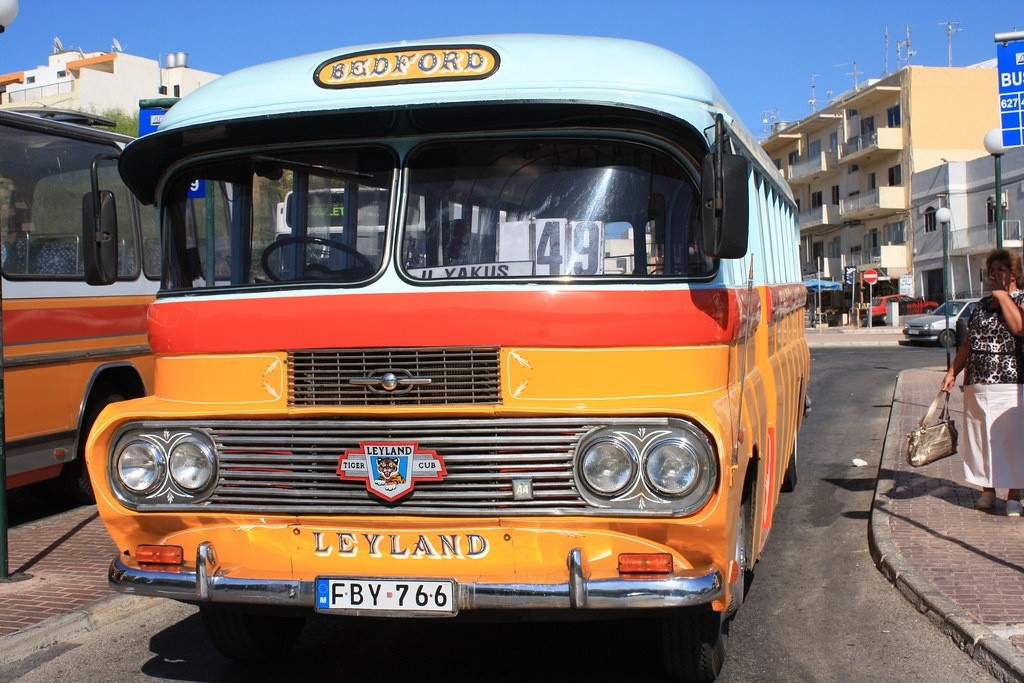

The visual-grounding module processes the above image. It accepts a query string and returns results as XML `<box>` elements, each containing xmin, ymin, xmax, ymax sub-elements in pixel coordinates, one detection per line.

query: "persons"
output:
<box><xmin>941</xmin><ymin>247</ymin><xmax>1024</xmax><ymax>517</ymax></box>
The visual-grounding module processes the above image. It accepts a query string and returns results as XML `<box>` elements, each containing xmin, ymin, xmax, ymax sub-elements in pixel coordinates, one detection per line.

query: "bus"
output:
<box><xmin>85</xmin><ymin>35</ymin><xmax>810</xmax><ymax>683</ymax></box>
<box><xmin>275</xmin><ymin>186</ymin><xmax>507</xmax><ymax>275</ymax></box>
<box><xmin>0</xmin><ymin>102</ymin><xmax>232</xmax><ymax>503</ymax></box>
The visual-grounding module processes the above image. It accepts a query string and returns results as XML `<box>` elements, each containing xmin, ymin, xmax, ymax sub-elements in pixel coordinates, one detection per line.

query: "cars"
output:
<box><xmin>859</xmin><ymin>294</ymin><xmax>938</xmax><ymax>326</ymax></box>
<box><xmin>903</xmin><ymin>297</ymin><xmax>984</xmax><ymax>349</ymax></box>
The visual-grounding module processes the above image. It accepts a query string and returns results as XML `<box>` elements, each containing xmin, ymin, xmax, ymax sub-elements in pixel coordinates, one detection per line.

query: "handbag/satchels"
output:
<box><xmin>906</xmin><ymin>389</ymin><xmax>959</xmax><ymax>467</ymax></box>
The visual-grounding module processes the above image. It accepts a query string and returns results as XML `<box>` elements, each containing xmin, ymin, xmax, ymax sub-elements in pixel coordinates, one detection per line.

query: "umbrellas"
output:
<box><xmin>803</xmin><ymin>279</ymin><xmax>842</xmax><ymax>324</ymax></box>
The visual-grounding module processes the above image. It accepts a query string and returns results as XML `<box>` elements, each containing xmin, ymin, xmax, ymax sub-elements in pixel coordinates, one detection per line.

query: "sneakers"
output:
<box><xmin>975</xmin><ymin>489</ymin><xmax>996</xmax><ymax>509</ymax></box>
<box><xmin>1006</xmin><ymin>500</ymin><xmax>1024</xmax><ymax>516</ymax></box>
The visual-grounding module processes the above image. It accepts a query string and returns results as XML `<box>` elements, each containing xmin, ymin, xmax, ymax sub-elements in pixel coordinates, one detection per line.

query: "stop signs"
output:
<box><xmin>864</xmin><ymin>269</ymin><xmax>878</xmax><ymax>283</ymax></box>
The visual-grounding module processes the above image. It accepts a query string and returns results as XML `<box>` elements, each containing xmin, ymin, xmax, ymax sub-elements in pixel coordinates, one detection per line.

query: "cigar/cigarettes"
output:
<box><xmin>990</xmin><ymin>276</ymin><xmax>994</xmax><ymax>279</ymax></box>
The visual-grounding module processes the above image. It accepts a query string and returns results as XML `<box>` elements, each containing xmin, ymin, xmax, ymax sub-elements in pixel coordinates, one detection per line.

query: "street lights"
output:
<box><xmin>935</xmin><ymin>206</ymin><xmax>953</xmax><ymax>373</ymax></box>
<box><xmin>983</xmin><ymin>130</ymin><xmax>1011</xmax><ymax>249</ymax></box>
